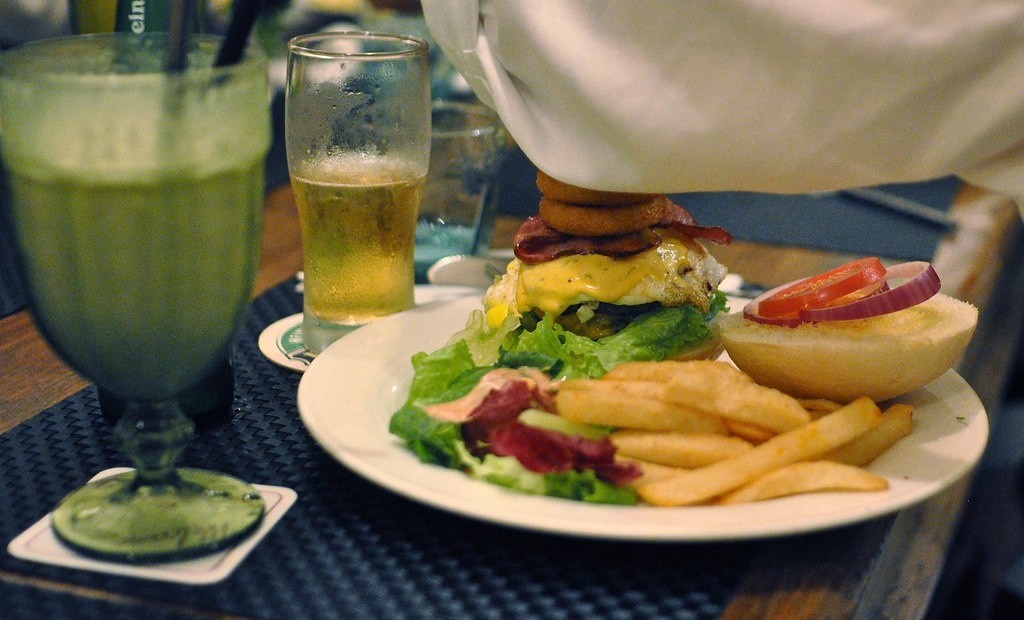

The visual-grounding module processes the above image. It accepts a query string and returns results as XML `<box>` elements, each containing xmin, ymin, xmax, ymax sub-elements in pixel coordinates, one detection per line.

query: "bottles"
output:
<box><xmin>96</xmin><ymin>342</ymin><xmax>239</xmax><ymax>442</ymax></box>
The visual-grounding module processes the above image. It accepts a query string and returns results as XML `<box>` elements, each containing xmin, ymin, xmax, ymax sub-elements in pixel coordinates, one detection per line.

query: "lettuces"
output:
<box><xmin>388</xmin><ymin>288</ymin><xmax>726</xmax><ymax>503</ymax></box>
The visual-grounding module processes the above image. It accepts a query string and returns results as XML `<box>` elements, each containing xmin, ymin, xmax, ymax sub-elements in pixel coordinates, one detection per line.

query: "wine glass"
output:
<box><xmin>1</xmin><ymin>32</ymin><xmax>275</xmax><ymax>560</ymax></box>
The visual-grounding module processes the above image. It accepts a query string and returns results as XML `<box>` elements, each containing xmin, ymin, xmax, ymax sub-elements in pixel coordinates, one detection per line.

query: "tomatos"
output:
<box><xmin>757</xmin><ymin>257</ymin><xmax>887</xmax><ymax>317</ymax></box>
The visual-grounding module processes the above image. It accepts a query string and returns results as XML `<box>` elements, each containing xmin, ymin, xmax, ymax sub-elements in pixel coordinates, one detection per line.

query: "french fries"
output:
<box><xmin>560</xmin><ymin>361</ymin><xmax>913</xmax><ymax>505</ymax></box>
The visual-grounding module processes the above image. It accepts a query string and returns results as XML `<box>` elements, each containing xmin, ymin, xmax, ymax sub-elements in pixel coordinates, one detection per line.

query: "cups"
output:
<box><xmin>283</xmin><ymin>31</ymin><xmax>432</xmax><ymax>353</ymax></box>
<box><xmin>409</xmin><ymin>100</ymin><xmax>507</xmax><ymax>285</ymax></box>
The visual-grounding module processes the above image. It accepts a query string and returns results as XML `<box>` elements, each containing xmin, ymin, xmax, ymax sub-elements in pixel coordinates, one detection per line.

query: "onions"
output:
<box><xmin>742</xmin><ymin>261</ymin><xmax>941</xmax><ymax>327</ymax></box>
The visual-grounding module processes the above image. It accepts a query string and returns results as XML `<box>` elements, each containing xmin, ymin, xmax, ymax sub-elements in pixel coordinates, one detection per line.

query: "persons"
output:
<box><xmin>421</xmin><ymin>1</ymin><xmax>1024</xmax><ymax>216</ymax></box>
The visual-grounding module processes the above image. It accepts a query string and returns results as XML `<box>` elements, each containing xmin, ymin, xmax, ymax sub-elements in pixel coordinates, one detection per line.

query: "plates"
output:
<box><xmin>294</xmin><ymin>293</ymin><xmax>990</xmax><ymax>543</ymax></box>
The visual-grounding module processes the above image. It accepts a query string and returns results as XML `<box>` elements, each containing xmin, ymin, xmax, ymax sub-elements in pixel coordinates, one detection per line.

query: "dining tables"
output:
<box><xmin>0</xmin><ymin>82</ymin><xmax>1012</xmax><ymax>620</ymax></box>
